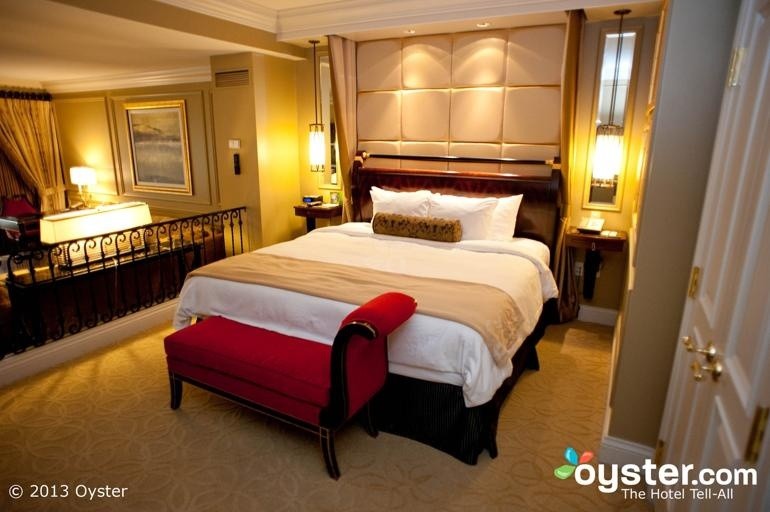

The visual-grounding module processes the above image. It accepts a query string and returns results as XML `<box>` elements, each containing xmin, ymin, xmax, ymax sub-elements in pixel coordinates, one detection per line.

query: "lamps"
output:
<box><xmin>591</xmin><ymin>8</ymin><xmax>634</xmax><ymax>187</ymax></box>
<box><xmin>304</xmin><ymin>38</ymin><xmax>328</xmax><ymax>175</ymax></box>
<box><xmin>39</xmin><ymin>202</ymin><xmax>153</xmax><ymax>268</ymax></box>
<box><xmin>68</xmin><ymin>165</ymin><xmax>97</xmax><ymax>207</ymax></box>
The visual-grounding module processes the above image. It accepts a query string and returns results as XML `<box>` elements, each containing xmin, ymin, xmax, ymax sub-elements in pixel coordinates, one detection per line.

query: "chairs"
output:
<box><xmin>1</xmin><ymin>192</ymin><xmax>44</xmax><ymax>244</ymax></box>
<box><xmin>163</xmin><ymin>291</ymin><xmax>418</xmax><ymax>481</ymax></box>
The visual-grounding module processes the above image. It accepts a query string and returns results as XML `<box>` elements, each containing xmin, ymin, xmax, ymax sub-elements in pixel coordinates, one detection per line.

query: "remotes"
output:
<box><xmin>308</xmin><ymin>201</ymin><xmax>322</xmax><ymax>207</ymax></box>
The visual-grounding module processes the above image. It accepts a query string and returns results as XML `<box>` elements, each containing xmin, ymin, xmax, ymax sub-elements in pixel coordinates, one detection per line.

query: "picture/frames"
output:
<box><xmin>122</xmin><ymin>97</ymin><xmax>194</xmax><ymax>199</ymax></box>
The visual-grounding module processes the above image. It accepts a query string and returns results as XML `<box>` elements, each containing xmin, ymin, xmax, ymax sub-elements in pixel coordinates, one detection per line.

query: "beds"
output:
<box><xmin>172</xmin><ymin>149</ymin><xmax>562</xmax><ymax>466</ymax></box>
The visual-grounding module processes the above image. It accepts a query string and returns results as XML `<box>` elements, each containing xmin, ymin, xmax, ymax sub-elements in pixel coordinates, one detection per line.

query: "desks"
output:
<box><xmin>563</xmin><ymin>225</ymin><xmax>626</xmax><ymax>300</ymax></box>
<box><xmin>293</xmin><ymin>200</ymin><xmax>342</xmax><ymax>232</ymax></box>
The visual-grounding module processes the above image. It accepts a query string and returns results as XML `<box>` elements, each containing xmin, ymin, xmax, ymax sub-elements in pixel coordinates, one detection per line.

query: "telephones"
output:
<box><xmin>578</xmin><ymin>217</ymin><xmax>605</xmax><ymax>234</ymax></box>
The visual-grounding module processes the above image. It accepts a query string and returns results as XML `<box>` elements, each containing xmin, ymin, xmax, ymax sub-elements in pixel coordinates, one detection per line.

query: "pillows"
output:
<box><xmin>368</xmin><ymin>185</ymin><xmax>525</xmax><ymax>242</ymax></box>
